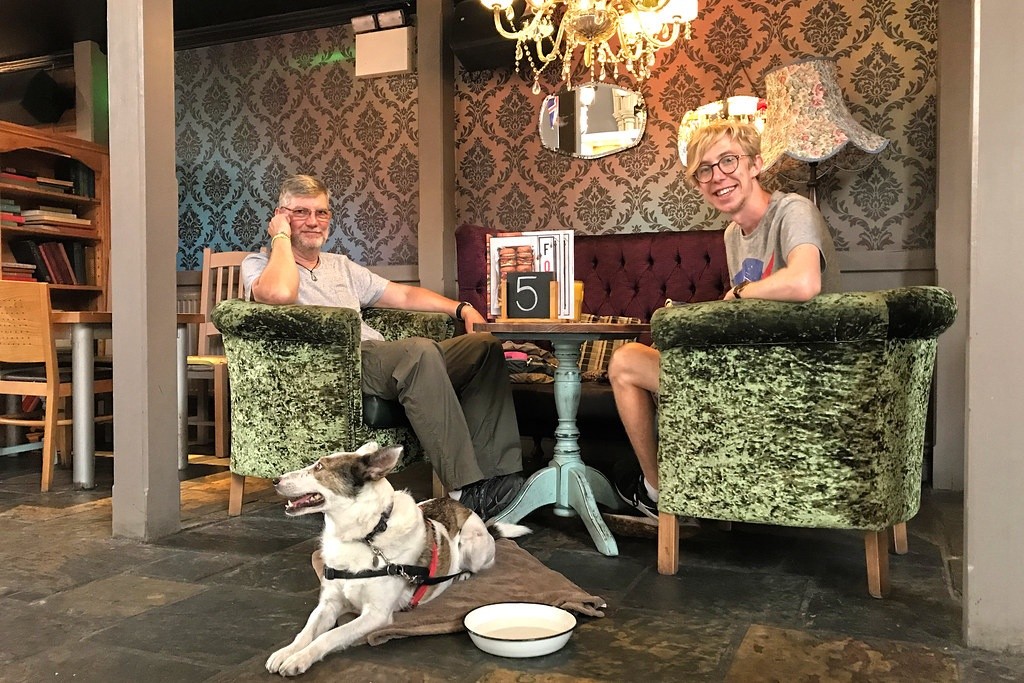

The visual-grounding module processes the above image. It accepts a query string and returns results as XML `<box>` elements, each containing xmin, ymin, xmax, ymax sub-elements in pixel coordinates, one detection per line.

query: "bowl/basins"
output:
<box><xmin>462</xmin><ymin>602</ymin><xmax>578</xmax><ymax>660</ymax></box>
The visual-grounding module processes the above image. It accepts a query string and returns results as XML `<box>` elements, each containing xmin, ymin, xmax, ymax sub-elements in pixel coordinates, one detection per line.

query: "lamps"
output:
<box><xmin>759</xmin><ymin>57</ymin><xmax>891</xmax><ymax>211</ymax></box>
<box><xmin>351</xmin><ymin>9</ymin><xmax>415</xmax><ymax>78</ymax></box>
<box><xmin>479</xmin><ymin>0</ymin><xmax>697</xmax><ymax>96</ymax></box>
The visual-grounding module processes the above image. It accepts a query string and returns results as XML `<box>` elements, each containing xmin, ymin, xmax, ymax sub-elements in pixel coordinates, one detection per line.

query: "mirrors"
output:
<box><xmin>538</xmin><ymin>82</ymin><xmax>647</xmax><ymax>160</ymax></box>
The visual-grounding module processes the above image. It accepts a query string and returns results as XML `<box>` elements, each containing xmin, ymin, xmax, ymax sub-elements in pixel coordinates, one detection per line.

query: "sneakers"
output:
<box><xmin>614</xmin><ymin>471</ymin><xmax>659</xmax><ymax>520</ymax></box>
<box><xmin>459</xmin><ymin>476</ymin><xmax>526</xmax><ymax>527</ymax></box>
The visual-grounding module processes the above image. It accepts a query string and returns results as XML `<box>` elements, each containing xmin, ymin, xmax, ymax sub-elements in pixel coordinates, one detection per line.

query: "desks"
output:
<box><xmin>473</xmin><ymin>322</ymin><xmax>650</xmax><ymax>557</ymax></box>
<box><xmin>5</xmin><ymin>312</ymin><xmax>206</xmax><ymax>489</ymax></box>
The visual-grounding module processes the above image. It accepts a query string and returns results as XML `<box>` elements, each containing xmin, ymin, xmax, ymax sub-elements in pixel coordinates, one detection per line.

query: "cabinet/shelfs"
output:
<box><xmin>1</xmin><ymin>120</ymin><xmax>111</xmax><ymax>356</ymax></box>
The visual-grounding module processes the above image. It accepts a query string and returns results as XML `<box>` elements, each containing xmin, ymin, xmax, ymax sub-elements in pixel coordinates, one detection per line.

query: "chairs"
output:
<box><xmin>650</xmin><ymin>286</ymin><xmax>958</xmax><ymax>599</ymax></box>
<box><xmin>0</xmin><ymin>280</ymin><xmax>113</xmax><ymax>492</ymax></box>
<box><xmin>187</xmin><ymin>247</ymin><xmax>271</xmax><ymax>458</ymax></box>
<box><xmin>211</xmin><ymin>298</ymin><xmax>455</xmax><ymax>517</ymax></box>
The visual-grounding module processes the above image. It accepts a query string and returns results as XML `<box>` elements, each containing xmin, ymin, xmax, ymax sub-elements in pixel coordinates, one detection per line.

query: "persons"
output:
<box><xmin>242</xmin><ymin>175</ymin><xmax>523</xmax><ymax>522</ymax></box>
<box><xmin>608</xmin><ymin>121</ymin><xmax>839</xmax><ymax>526</ymax></box>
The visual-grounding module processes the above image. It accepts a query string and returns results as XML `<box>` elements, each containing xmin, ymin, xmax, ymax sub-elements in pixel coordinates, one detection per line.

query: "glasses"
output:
<box><xmin>694</xmin><ymin>155</ymin><xmax>756</xmax><ymax>182</ymax></box>
<box><xmin>281</xmin><ymin>206</ymin><xmax>333</xmax><ymax>220</ymax></box>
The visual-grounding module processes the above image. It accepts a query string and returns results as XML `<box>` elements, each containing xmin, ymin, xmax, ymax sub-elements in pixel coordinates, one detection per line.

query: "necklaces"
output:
<box><xmin>296</xmin><ymin>256</ymin><xmax>319</xmax><ymax>281</ymax></box>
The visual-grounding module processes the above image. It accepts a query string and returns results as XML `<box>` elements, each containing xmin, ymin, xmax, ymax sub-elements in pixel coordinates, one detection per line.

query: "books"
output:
<box><xmin>0</xmin><ymin>166</ymin><xmax>95</xmax><ymax>286</ymax></box>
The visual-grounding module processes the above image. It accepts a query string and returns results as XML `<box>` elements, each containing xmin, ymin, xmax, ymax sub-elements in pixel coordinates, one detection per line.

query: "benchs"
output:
<box><xmin>455</xmin><ymin>224</ymin><xmax>732</xmax><ymax>418</ymax></box>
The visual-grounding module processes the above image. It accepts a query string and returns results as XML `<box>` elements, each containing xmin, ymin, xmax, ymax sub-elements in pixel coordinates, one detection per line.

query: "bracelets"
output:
<box><xmin>270</xmin><ymin>232</ymin><xmax>290</xmax><ymax>246</ymax></box>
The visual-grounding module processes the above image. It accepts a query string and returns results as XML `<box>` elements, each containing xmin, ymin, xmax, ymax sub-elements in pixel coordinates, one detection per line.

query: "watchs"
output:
<box><xmin>733</xmin><ymin>281</ymin><xmax>750</xmax><ymax>299</ymax></box>
<box><xmin>456</xmin><ymin>301</ymin><xmax>473</xmax><ymax>323</ymax></box>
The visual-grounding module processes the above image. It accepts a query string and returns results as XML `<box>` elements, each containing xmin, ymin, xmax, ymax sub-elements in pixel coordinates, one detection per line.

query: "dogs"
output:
<box><xmin>264</xmin><ymin>442</ymin><xmax>533</xmax><ymax>677</ymax></box>
